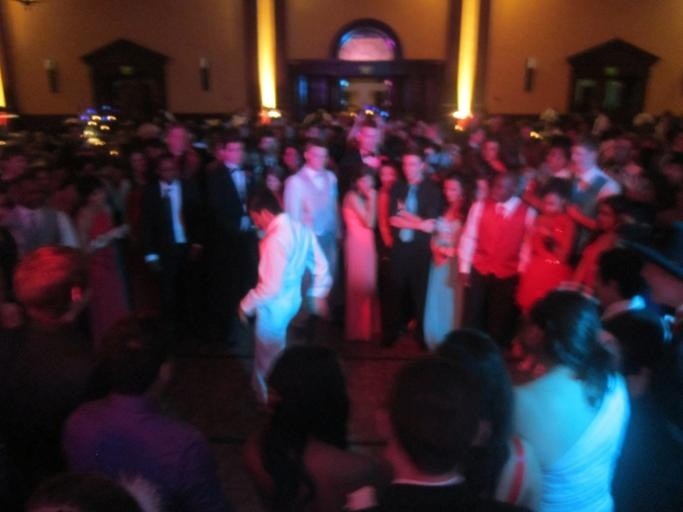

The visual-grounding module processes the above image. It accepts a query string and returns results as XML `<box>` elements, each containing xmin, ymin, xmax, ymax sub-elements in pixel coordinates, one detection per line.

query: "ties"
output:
<box><xmin>400</xmin><ymin>187</ymin><xmax>416</xmax><ymax>243</ymax></box>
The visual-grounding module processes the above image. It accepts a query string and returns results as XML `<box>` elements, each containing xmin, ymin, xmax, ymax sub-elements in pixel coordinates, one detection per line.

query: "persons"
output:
<box><xmin>0</xmin><ymin>108</ymin><xmax>682</xmax><ymax>512</ymax></box>
